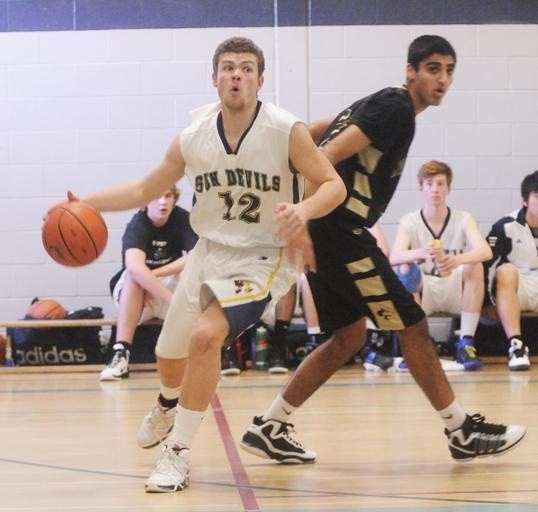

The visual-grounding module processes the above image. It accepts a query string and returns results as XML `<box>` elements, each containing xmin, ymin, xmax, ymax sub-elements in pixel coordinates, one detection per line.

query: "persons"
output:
<box><xmin>43</xmin><ymin>37</ymin><xmax>348</xmax><ymax>491</ymax></box>
<box><xmin>387</xmin><ymin>160</ymin><xmax>494</xmax><ymax>371</ymax></box>
<box><xmin>99</xmin><ymin>184</ymin><xmax>200</xmax><ymax>379</ymax></box>
<box><xmin>486</xmin><ymin>170</ymin><xmax>538</xmax><ymax>371</ymax></box>
<box><xmin>236</xmin><ymin>36</ymin><xmax>527</xmax><ymax>466</ymax></box>
<box><xmin>300</xmin><ymin>275</ymin><xmax>321</xmax><ymax>350</ymax></box>
<box><xmin>220</xmin><ymin>279</ymin><xmax>296</xmax><ymax>373</ymax></box>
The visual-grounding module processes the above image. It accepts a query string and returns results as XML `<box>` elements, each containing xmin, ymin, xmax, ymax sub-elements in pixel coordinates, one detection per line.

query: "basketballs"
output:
<box><xmin>26</xmin><ymin>300</ymin><xmax>66</xmax><ymax>319</ymax></box>
<box><xmin>42</xmin><ymin>202</ymin><xmax>108</xmax><ymax>268</ymax></box>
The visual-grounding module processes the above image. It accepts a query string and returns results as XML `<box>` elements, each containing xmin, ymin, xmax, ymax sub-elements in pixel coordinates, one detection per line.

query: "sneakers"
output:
<box><xmin>99</xmin><ymin>342</ymin><xmax>133</xmax><ymax>381</ymax></box>
<box><xmin>239</xmin><ymin>415</ymin><xmax>317</xmax><ymax>464</ymax></box>
<box><xmin>443</xmin><ymin>413</ymin><xmax>528</xmax><ymax>462</ymax></box>
<box><xmin>358</xmin><ymin>329</ymin><xmax>394</xmax><ymax>372</ymax></box>
<box><xmin>220</xmin><ymin>345</ymin><xmax>241</xmax><ymax>375</ymax></box>
<box><xmin>399</xmin><ymin>359</ymin><xmax>408</xmax><ymax>368</ymax></box>
<box><xmin>454</xmin><ymin>336</ymin><xmax>484</xmax><ymax>370</ymax></box>
<box><xmin>136</xmin><ymin>397</ymin><xmax>177</xmax><ymax>449</ymax></box>
<box><xmin>267</xmin><ymin>331</ymin><xmax>289</xmax><ymax>373</ymax></box>
<box><xmin>294</xmin><ymin>334</ymin><xmax>320</xmax><ymax>367</ymax></box>
<box><xmin>507</xmin><ymin>337</ymin><xmax>530</xmax><ymax>371</ymax></box>
<box><xmin>144</xmin><ymin>439</ymin><xmax>191</xmax><ymax>493</ymax></box>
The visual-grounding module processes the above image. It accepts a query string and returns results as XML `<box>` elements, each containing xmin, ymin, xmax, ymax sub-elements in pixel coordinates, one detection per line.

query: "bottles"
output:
<box><xmin>254</xmin><ymin>324</ymin><xmax>269</xmax><ymax>371</ymax></box>
<box><xmin>430</xmin><ymin>240</ymin><xmax>452</xmax><ymax>278</ymax></box>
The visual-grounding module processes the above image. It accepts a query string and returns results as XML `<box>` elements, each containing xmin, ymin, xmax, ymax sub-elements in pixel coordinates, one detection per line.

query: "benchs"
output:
<box><xmin>421</xmin><ymin>311</ymin><xmax>537</xmax><ymax>365</ymax></box>
<box><xmin>1</xmin><ymin>317</ymin><xmax>164</xmax><ymax>375</ymax></box>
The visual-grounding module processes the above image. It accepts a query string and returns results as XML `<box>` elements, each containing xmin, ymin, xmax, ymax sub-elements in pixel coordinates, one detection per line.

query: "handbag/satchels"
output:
<box><xmin>66</xmin><ymin>306</ymin><xmax>104</xmax><ymax>319</ymax></box>
